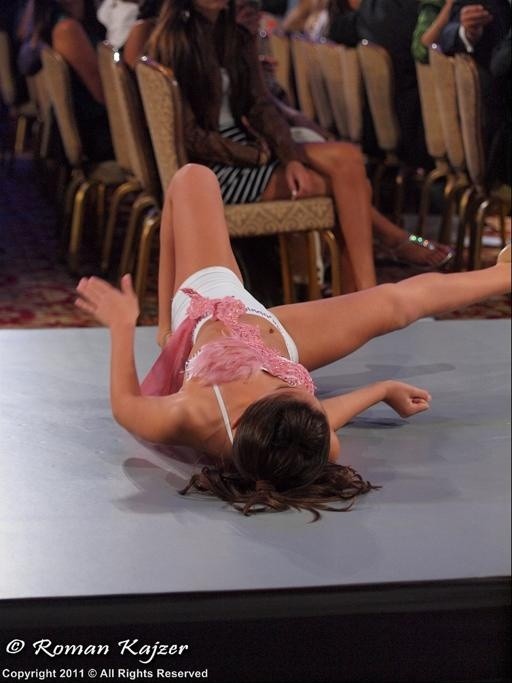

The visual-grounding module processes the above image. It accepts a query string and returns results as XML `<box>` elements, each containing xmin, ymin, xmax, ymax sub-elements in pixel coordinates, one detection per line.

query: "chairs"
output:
<box><xmin>0</xmin><ymin>23</ymin><xmax>511</xmax><ymax>325</ymax></box>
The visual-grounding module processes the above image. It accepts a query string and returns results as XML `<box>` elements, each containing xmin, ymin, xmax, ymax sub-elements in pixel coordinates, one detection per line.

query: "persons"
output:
<box><xmin>0</xmin><ymin>1</ymin><xmax>511</xmax><ymax>270</ymax></box>
<box><xmin>143</xmin><ymin>0</ymin><xmax>455</xmax><ymax>293</ymax></box>
<box><xmin>76</xmin><ymin>159</ymin><xmax>511</xmax><ymax>511</ymax></box>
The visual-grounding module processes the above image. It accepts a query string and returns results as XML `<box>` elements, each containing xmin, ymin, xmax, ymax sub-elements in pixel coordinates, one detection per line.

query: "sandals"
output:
<box><xmin>376</xmin><ymin>236</ymin><xmax>454</xmax><ymax>268</ymax></box>
